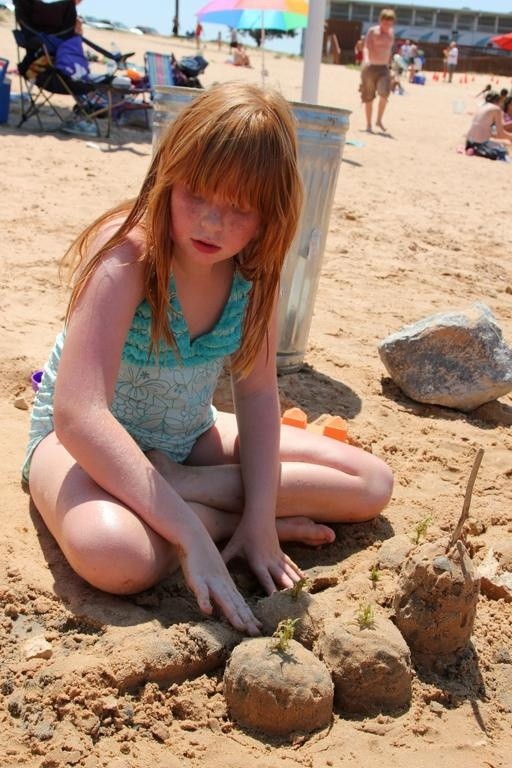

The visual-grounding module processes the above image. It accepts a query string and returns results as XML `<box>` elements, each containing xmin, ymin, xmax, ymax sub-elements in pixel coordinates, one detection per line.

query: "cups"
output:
<box><xmin>30</xmin><ymin>371</ymin><xmax>43</xmax><ymax>394</ymax></box>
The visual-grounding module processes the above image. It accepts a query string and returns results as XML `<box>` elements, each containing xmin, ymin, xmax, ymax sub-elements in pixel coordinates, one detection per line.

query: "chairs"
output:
<box><xmin>12</xmin><ymin>0</ymin><xmax>176</xmax><ymax>139</ymax></box>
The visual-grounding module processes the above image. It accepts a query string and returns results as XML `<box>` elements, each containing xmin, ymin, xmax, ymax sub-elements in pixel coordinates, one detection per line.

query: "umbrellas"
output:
<box><xmin>196</xmin><ymin>1</ymin><xmax>310</xmax><ymax>93</ymax></box>
<box><xmin>490</xmin><ymin>33</ymin><xmax>512</xmax><ymax>53</ymax></box>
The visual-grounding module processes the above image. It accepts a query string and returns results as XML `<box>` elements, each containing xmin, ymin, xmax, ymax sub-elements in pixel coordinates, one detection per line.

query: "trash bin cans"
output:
<box><xmin>150</xmin><ymin>85</ymin><xmax>352</xmax><ymax>374</ymax></box>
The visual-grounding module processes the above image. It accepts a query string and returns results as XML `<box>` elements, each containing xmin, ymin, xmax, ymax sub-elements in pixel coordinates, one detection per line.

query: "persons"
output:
<box><xmin>358</xmin><ymin>7</ymin><xmax>390</xmax><ymax>133</ymax></box>
<box><xmin>16</xmin><ymin>0</ymin><xmax>101</xmax><ymax>136</ymax></box>
<box><xmin>465</xmin><ymin>84</ymin><xmax>512</xmax><ymax>161</ymax></box>
<box><xmin>20</xmin><ymin>85</ymin><xmax>395</xmax><ymax>638</ymax></box>
<box><xmin>171</xmin><ymin>16</ymin><xmax>250</xmax><ymax>67</ymax></box>
<box><xmin>326</xmin><ymin>29</ymin><xmax>459</xmax><ymax>84</ymax></box>
